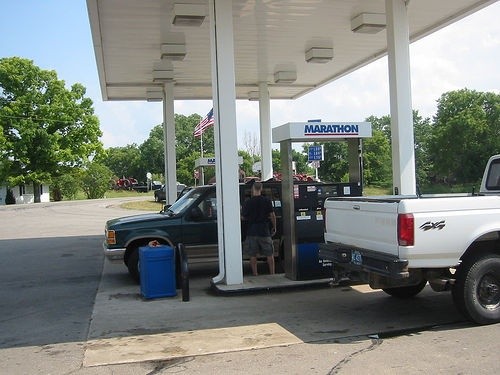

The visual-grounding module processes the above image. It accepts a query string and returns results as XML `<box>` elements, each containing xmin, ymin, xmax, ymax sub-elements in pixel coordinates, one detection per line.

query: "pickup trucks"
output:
<box><xmin>318</xmin><ymin>154</ymin><xmax>499</xmax><ymax>325</ymax></box>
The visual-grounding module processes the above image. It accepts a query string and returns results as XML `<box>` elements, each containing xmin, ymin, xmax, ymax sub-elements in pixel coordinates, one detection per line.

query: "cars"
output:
<box><xmin>154</xmin><ymin>183</ymin><xmax>188</xmax><ymax>202</ymax></box>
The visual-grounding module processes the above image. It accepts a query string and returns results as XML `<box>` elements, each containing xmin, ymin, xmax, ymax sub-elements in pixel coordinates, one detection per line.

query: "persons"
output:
<box><xmin>241</xmin><ymin>182</ymin><xmax>277</xmax><ymax>277</ymax></box>
<box><xmin>117</xmin><ymin>176</ymin><xmax>137</xmax><ymax>191</ymax></box>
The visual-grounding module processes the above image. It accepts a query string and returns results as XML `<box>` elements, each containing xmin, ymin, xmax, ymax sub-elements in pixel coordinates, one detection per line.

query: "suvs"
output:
<box><xmin>102</xmin><ymin>178</ymin><xmax>331</xmax><ymax>284</ymax></box>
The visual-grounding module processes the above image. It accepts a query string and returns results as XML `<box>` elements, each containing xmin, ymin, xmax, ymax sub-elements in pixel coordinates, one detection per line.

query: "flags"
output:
<box><xmin>194</xmin><ymin>108</ymin><xmax>214</xmax><ymax>137</ymax></box>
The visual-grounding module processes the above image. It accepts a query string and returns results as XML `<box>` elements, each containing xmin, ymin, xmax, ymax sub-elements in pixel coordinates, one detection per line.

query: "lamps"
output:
<box><xmin>351</xmin><ymin>14</ymin><xmax>386</xmax><ymax>35</ymax></box>
<box><xmin>306</xmin><ymin>48</ymin><xmax>333</xmax><ymax>64</ymax></box>
<box><xmin>247</xmin><ymin>91</ymin><xmax>259</xmax><ymax>101</ymax></box>
<box><xmin>153</xmin><ymin>70</ymin><xmax>173</xmax><ymax>83</ymax></box>
<box><xmin>147</xmin><ymin>91</ymin><xmax>162</xmax><ymax>102</ymax></box>
<box><xmin>171</xmin><ymin>4</ymin><xmax>206</xmax><ymax>27</ymax></box>
<box><xmin>274</xmin><ymin>71</ymin><xmax>297</xmax><ymax>84</ymax></box>
<box><xmin>161</xmin><ymin>44</ymin><xmax>186</xmax><ymax>60</ymax></box>
